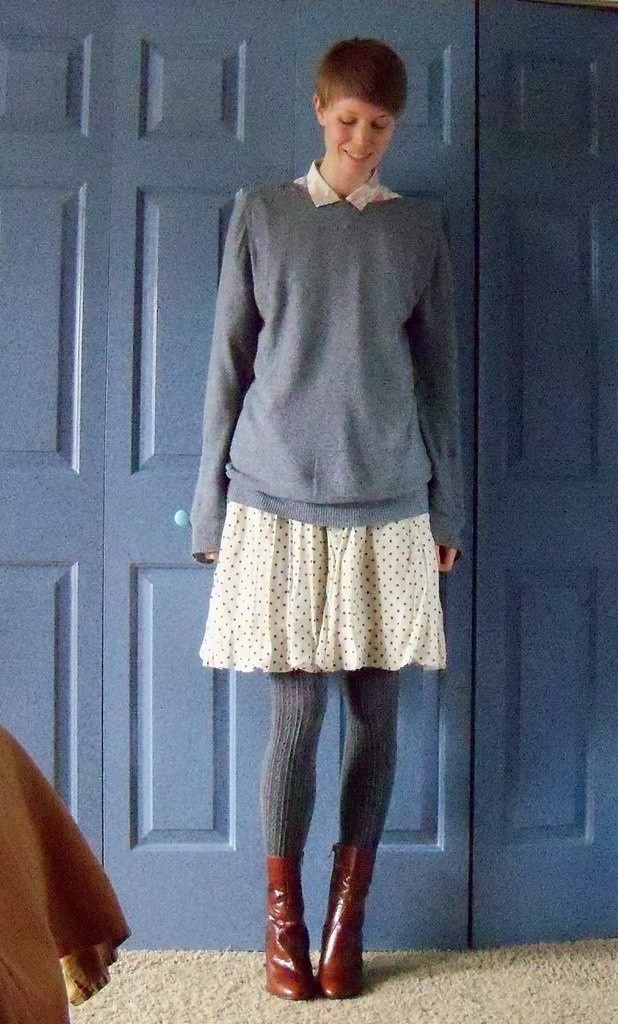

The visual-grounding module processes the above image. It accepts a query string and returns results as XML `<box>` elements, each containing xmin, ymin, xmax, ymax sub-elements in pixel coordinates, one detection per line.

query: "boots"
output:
<box><xmin>315</xmin><ymin>843</ymin><xmax>376</xmax><ymax>999</ymax></box>
<box><xmin>265</xmin><ymin>850</ymin><xmax>315</xmax><ymax>999</ymax></box>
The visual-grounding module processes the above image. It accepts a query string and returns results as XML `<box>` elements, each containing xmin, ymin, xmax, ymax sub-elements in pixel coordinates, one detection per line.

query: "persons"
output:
<box><xmin>189</xmin><ymin>36</ymin><xmax>465</xmax><ymax>998</ymax></box>
